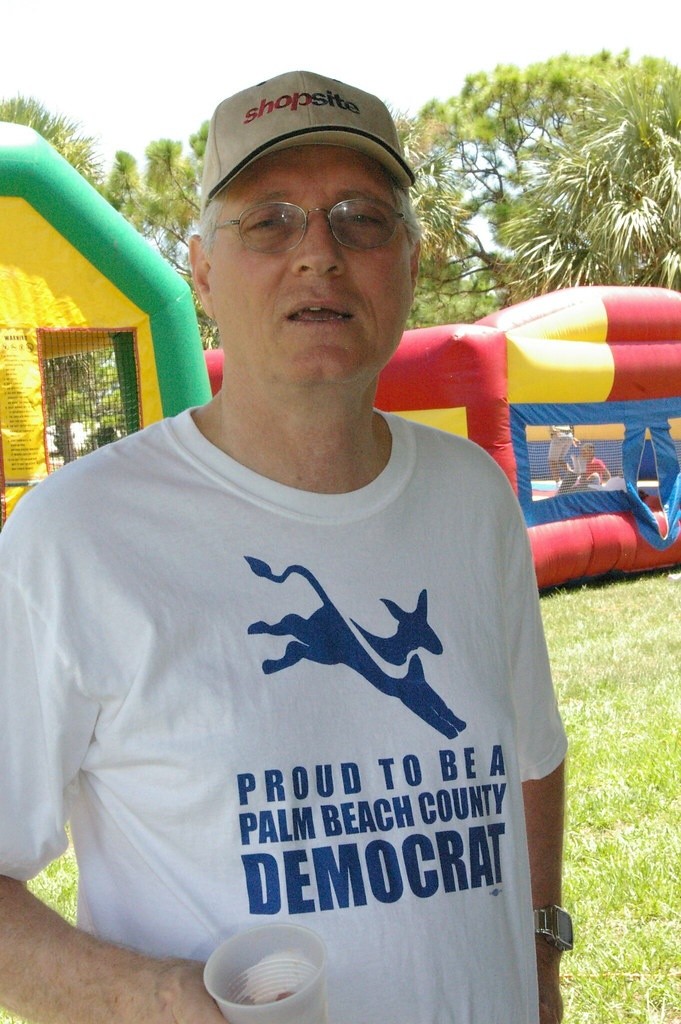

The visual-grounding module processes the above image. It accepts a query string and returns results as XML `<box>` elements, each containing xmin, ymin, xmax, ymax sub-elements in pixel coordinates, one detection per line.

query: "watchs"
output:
<box><xmin>534</xmin><ymin>906</ymin><xmax>576</xmax><ymax>952</ymax></box>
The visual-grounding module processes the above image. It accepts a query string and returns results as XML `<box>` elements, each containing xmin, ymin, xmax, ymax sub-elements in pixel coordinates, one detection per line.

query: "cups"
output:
<box><xmin>202</xmin><ymin>922</ymin><xmax>329</xmax><ymax>1024</ymax></box>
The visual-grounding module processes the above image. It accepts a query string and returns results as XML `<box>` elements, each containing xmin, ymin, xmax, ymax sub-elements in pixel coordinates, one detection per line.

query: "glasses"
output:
<box><xmin>214</xmin><ymin>197</ymin><xmax>405</xmax><ymax>254</ymax></box>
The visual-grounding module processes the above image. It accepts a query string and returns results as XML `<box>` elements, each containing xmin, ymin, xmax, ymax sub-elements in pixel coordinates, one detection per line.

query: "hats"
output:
<box><xmin>200</xmin><ymin>71</ymin><xmax>415</xmax><ymax>218</ymax></box>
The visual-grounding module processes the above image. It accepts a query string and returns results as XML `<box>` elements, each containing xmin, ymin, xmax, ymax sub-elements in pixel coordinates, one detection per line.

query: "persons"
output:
<box><xmin>547</xmin><ymin>425</ymin><xmax>627</xmax><ymax>497</ymax></box>
<box><xmin>0</xmin><ymin>68</ymin><xmax>573</xmax><ymax>1024</ymax></box>
